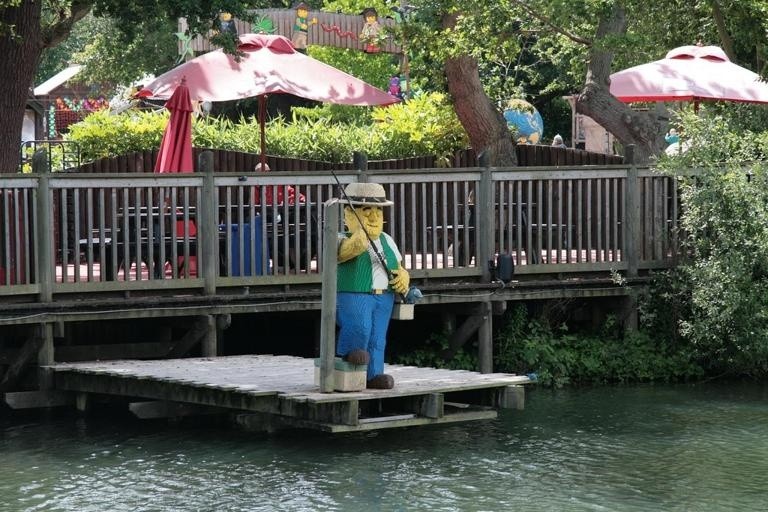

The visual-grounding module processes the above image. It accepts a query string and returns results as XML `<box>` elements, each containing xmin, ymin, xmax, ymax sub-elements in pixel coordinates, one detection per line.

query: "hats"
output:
<box><xmin>255</xmin><ymin>162</ymin><xmax>270</xmax><ymax>173</ymax></box>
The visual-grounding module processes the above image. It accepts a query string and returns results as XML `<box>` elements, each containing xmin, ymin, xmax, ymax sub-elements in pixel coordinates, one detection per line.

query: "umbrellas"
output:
<box><xmin>608</xmin><ymin>44</ymin><xmax>768</xmax><ymax>102</ymax></box>
<box><xmin>132</xmin><ymin>30</ymin><xmax>402</xmax><ymax>172</ymax></box>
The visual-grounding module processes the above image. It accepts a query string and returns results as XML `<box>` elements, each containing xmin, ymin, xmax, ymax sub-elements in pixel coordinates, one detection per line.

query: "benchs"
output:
<box><xmin>424</xmin><ymin>222</ymin><xmax>576</xmax><ymax>264</ymax></box>
<box><xmin>78</xmin><ymin>222</ymin><xmax>307</xmax><ymax>281</ymax></box>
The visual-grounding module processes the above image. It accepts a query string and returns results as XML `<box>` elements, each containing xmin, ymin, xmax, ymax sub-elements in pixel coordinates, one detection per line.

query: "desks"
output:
<box><xmin>454</xmin><ymin>203</ymin><xmax>545</xmax><ymax>266</ymax></box>
<box><xmin>101</xmin><ymin>202</ymin><xmax>324</xmax><ymax>282</ymax></box>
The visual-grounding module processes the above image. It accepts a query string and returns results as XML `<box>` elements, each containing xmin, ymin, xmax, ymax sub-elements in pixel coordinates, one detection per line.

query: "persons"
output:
<box><xmin>248</xmin><ymin>163</ymin><xmax>305</xmax><ymax>204</ymax></box>
<box><xmin>336</xmin><ymin>183</ymin><xmax>410</xmax><ymax>389</ymax></box>
